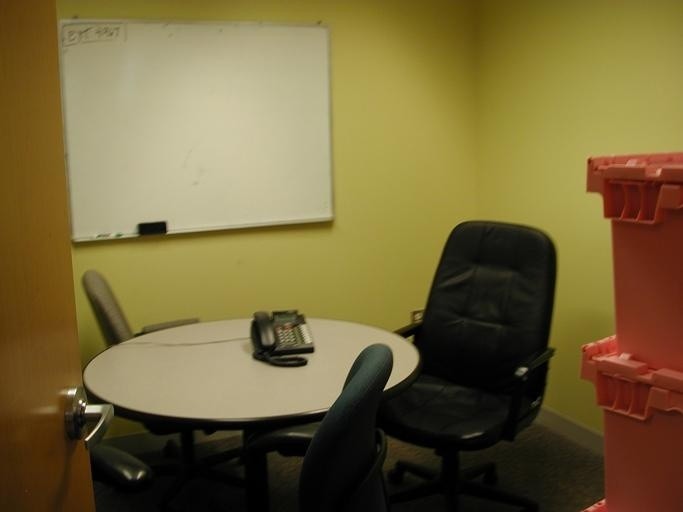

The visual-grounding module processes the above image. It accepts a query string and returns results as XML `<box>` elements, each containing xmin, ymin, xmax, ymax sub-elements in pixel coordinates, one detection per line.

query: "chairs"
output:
<box><xmin>372</xmin><ymin>219</ymin><xmax>557</xmax><ymax>510</ymax></box>
<box><xmin>232</xmin><ymin>342</ymin><xmax>393</xmax><ymax>512</ymax></box>
<box><xmin>80</xmin><ymin>270</ymin><xmax>228</xmax><ymax>512</ymax></box>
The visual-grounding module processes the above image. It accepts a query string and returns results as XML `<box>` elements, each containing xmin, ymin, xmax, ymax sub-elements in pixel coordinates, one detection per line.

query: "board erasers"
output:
<box><xmin>137</xmin><ymin>221</ymin><xmax>167</xmax><ymax>235</ymax></box>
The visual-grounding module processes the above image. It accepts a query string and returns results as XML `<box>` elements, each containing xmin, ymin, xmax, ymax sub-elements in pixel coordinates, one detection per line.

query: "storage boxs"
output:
<box><xmin>579</xmin><ymin>152</ymin><xmax>682</xmax><ymax>512</ymax></box>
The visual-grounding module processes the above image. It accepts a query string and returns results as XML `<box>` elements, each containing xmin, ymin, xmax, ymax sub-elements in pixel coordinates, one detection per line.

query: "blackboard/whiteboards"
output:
<box><xmin>57</xmin><ymin>18</ymin><xmax>334</xmax><ymax>243</ymax></box>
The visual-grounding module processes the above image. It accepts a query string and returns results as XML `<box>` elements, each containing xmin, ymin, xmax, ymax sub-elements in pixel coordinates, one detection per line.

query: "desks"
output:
<box><xmin>83</xmin><ymin>316</ymin><xmax>421</xmax><ymax>512</ymax></box>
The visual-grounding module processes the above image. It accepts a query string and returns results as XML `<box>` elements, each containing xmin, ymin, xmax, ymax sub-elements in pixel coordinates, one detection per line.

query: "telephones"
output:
<box><xmin>251</xmin><ymin>310</ymin><xmax>314</xmax><ymax>356</ymax></box>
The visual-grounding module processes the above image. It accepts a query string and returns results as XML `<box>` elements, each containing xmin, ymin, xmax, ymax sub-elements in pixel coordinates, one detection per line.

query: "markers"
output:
<box><xmin>97</xmin><ymin>234</ymin><xmax>122</xmax><ymax>238</ymax></box>
